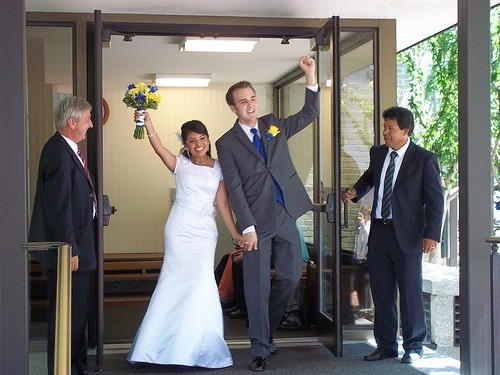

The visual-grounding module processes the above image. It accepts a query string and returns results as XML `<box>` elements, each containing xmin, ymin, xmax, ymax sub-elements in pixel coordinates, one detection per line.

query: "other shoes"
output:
<box><xmin>231</xmin><ymin>310</ymin><xmax>247</xmax><ymax>317</ymax></box>
<box><xmin>223</xmin><ymin>306</ymin><xmax>236</xmax><ymax>314</ymax></box>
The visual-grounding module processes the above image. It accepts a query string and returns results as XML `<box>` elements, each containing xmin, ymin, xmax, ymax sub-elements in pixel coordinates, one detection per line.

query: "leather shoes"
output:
<box><xmin>364</xmin><ymin>347</ymin><xmax>398</xmax><ymax>361</ymax></box>
<box><xmin>79</xmin><ymin>367</ymin><xmax>104</xmax><ymax>375</ymax></box>
<box><xmin>401</xmin><ymin>348</ymin><xmax>422</xmax><ymax>363</ymax></box>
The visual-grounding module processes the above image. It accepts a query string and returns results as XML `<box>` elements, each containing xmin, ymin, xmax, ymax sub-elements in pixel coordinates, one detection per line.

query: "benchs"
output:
<box><xmin>28</xmin><ymin>252</ymin><xmax>164</xmax><ymax>282</ymax></box>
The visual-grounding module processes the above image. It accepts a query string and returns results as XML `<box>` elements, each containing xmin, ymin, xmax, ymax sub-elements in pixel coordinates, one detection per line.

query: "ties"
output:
<box><xmin>381</xmin><ymin>152</ymin><xmax>398</xmax><ymax>224</ymax></box>
<box><xmin>77</xmin><ymin>146</ymin><xmax>98</xmax><ymax>214</ymax></box>
<box><xmin>250</xmin><ymin>128</ymin><xmax>284</xmax><ymax>206</ymax></box>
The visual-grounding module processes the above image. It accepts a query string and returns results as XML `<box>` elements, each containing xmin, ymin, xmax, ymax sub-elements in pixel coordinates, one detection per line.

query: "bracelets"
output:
<box><xmin>148</xmin><ymin>131</ymin><xmax>155</xmax><ymax>137</ymax></box>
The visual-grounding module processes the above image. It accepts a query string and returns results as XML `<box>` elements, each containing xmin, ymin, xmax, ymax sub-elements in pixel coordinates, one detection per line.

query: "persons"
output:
<box><xmin>353</xmin><ymin>205</ymin><xmax>372</xmax><ymax>318</ymax></box>
<box><xmin>340</xmin><ymin>106</ymin><xmax>444</xmax><ymax>363</ymax></box>
<box><xmin>127</xmin><ymin>110</ymin><xmax>246</xmax><ymax>368</ymax></box>
<box><xmin>26</xmin><ymin>97</ymin><xmax>104</xmax><ymax>375</ymax></box>
<box><xmin>215</xmin><ymin>57</ymin><xmax>320</xmax><ymax>372</ymax></box>
<box><xmin>214</xmin><ymin>220</ymin><xmax>247</xmax><ymax>318</ymax></box>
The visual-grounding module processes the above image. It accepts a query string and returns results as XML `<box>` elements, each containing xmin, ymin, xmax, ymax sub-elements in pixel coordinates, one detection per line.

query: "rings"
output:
<box><xmin>432</xmin><ymin>247</ymin><xmax>435</xmax><ymax>249</ymax></box>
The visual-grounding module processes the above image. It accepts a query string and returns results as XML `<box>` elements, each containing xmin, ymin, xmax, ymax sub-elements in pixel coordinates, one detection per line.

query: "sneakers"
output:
<box><xmin>268</xmin><ymin>336</ymin><xmax>278</xmax><ymax>354</ymax></box>
<box><xmin>249</xmin><ymin>355</ymin><xmax>266</xmax><ymax>371</ymax></box>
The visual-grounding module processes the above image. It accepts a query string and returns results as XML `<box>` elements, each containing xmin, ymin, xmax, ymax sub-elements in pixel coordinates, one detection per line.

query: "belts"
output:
<box><xmin>374</xmin><ymin>218</ymin><xmax>394</xmax><ymax>225</ymax></box>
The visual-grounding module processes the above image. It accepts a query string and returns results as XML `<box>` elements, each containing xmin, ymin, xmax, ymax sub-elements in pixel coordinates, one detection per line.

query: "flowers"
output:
<box><xmin>265</xmin><ymin>125</ymin><xmax>281</xmax><ymax>142</ymax></box>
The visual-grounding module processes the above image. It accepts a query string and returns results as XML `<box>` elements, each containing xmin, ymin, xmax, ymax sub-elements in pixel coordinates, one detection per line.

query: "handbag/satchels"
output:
<box><xmin>217</xmin><ymin>255</ymin><xmax>234</xmax><ymax>303</ymax></box>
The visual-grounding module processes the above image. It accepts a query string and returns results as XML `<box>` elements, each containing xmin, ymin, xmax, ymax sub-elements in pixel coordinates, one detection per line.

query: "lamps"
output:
<box><xmin>152</xmin><ymin>73</ymin><xmax>212</xmax><ymax>88</ymax></box>
<box><xmin>122</xmin><ymin>82</ymin><xmax>161</xmax><ymax>140</ymax></box>
<box><xmin>178</xmin><ymin>36</ymin><xmax>259</xmax><ymax>53</ymax></box>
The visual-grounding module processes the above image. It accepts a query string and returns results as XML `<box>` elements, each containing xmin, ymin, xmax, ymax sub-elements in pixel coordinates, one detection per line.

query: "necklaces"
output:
<box><xmin>192</xmin><ymin>155</ymin><xmax>210</xmax><ymax>165</ymax></box>
